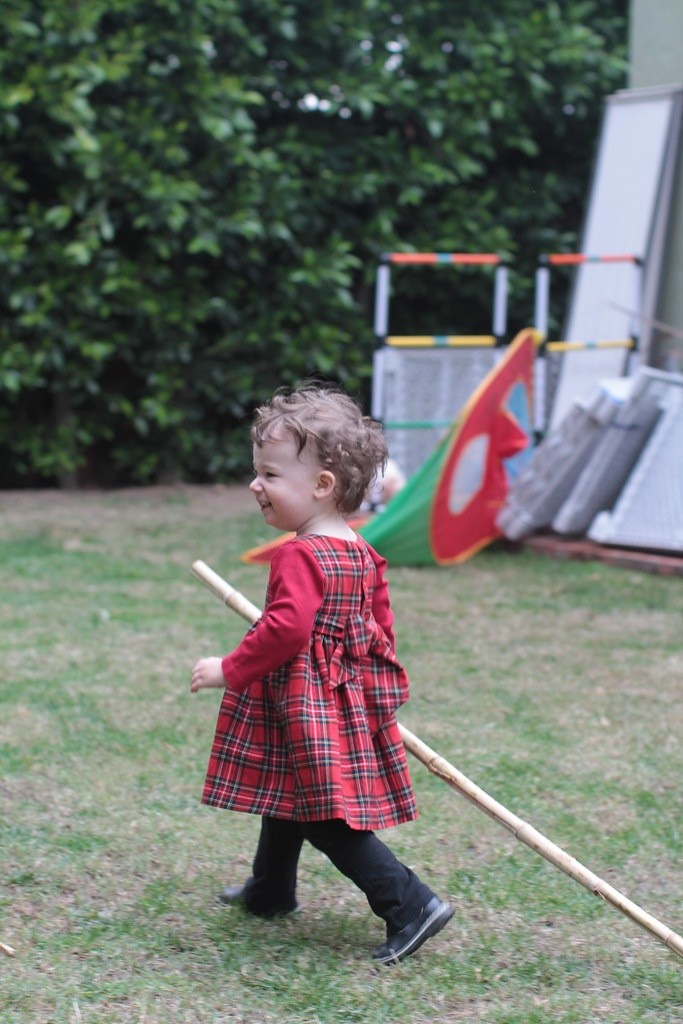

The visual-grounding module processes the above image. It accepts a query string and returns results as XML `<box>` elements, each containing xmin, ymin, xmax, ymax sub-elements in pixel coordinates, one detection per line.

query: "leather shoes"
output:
<box><xmin>372</xmin><ymin>895</ymin><xmax>455</xmax><ymax>966</ymax></box>
<box><xmin>220</xmin><ymin>886</ymin><xmax>298</xmax><ymax>920</ymax></box>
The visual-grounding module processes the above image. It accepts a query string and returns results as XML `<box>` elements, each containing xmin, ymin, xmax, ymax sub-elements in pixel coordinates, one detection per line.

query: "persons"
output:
<box><xmin>189</xmin><ymin>386</ymin><xmax>453</xmax><ymax>965</ymax></box>
<box><xmin>358</xmin><ymin>456</ymin><xmax>405</xmax><ymax>513</ymax></box>
